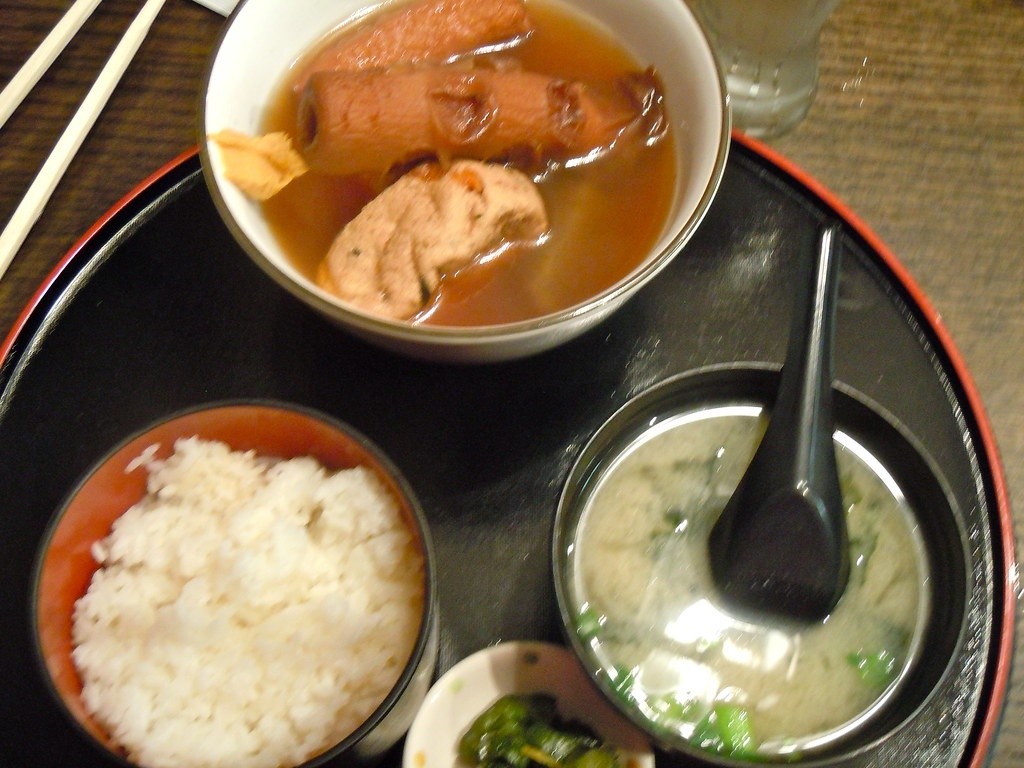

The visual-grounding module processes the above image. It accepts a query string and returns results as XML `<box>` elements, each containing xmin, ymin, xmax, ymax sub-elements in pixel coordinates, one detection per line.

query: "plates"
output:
<box><xmin>2</xmin><ymin>131</ymin><xmax>1015</xmax><ymax>768</ymax></box>
<box><xmin>394</xmin><ymin>638</ymin><xmax>660</xmax><ymax>768</ymax></box>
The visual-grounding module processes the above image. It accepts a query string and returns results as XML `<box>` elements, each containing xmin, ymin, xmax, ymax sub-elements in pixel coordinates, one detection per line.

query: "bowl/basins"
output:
<box><xmin>195</xmin><ymin>0</ymin><xmax>735</xmax><ymax>367</ymax></box>
<box><xmin>27</xmin><ymin>396</ymin><xmax>439</xmax><ymax>768</ymax></box>
<box><xmin>549</xmin><ymin>362</ymin><xmax>972</xmax><ymax>768</ymax></box>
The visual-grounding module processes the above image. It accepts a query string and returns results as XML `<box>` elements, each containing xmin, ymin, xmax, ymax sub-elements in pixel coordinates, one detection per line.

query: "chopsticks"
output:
<box><xmin>0</xmin><ymin>0</ymin><xmax>171</xmax><ymax>287</ymax></box>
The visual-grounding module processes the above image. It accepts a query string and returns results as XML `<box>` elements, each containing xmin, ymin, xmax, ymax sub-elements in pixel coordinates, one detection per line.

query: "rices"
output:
<box><xmin>64</xmin><ymin>432</ymin><xmax>423</xmax><ymax>768</ymax></box>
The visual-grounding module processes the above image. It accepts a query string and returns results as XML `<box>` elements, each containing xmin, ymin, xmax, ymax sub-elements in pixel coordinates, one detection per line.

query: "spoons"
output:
<box><xmin>706</xmin><ymin>221</ymin><xmax>854</xmax><ymax>626</ymax></box>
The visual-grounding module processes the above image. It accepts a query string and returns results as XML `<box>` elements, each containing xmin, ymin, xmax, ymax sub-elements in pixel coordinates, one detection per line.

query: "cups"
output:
<box><xmin>682</xmin><ymin>0</ymin><xmax>824</xmax><ymax>143</ymax></box>
